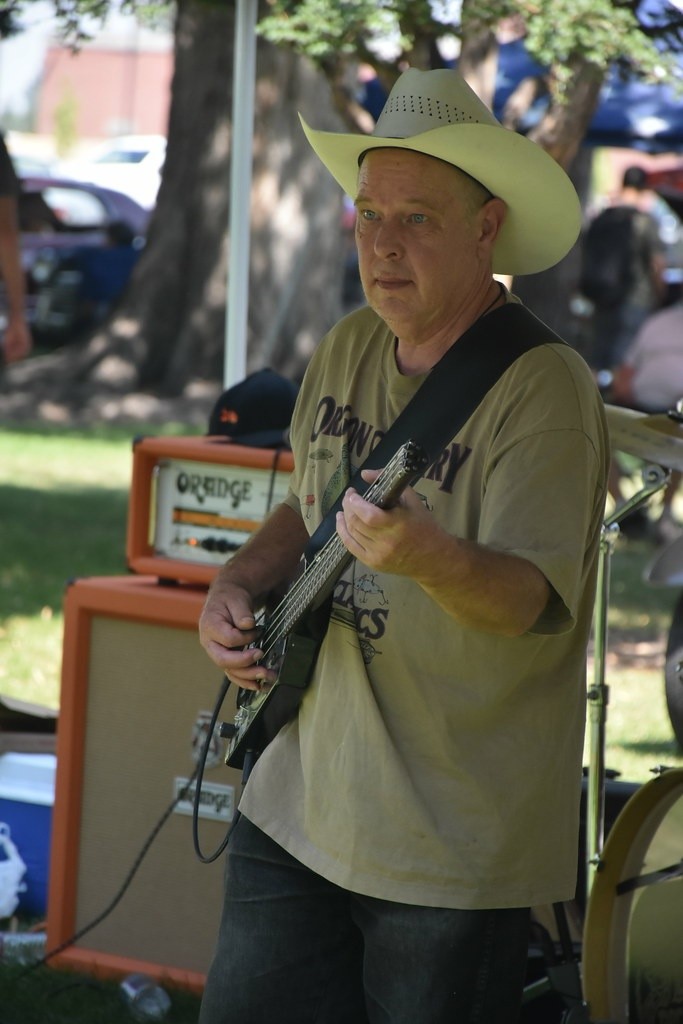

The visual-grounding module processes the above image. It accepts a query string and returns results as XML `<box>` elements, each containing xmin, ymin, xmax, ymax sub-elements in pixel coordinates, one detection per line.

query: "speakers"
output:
<box><xmin>48</xmin><ymin>576</ymin><xmax>248</xmax><ymax>998</ymax></box>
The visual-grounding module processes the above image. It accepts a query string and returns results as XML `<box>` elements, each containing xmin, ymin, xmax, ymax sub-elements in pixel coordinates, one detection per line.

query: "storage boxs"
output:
<box><xmin>0</xmin><ymin>752</ymin><xmax>55</xmax><ymax>914</ymax></box>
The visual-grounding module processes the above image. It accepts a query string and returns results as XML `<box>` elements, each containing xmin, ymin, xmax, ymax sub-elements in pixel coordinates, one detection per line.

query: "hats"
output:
<box><xmin>207</xmin><ymin>370</ymin><xmax>301</xmax><ymax>437</ymax></box>
<box><xmin>295</xmin><ymin>68</ymin><xmax>581</xmax><ymax>275</ymax></box>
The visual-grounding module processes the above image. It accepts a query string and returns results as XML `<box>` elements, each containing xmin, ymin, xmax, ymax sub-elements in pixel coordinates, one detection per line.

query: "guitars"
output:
<box><xmin>218</xmin><ymin>438</ymin><xmax>431</xmax><ymax>770</ymax></box>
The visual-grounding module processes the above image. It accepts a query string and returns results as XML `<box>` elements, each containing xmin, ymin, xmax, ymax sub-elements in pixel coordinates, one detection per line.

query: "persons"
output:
<box><xmin>194</xmin><ymin>65</ymin><xmax>613</xmax><ymax>1024</ymax></box>
<box><xmin>0</xmin><ymin>138</ymin><xmax>29</xmax><ymax>389</ymax></box>
<box><xmin>332</xmin><ymin>7</ymin><xmax>682</xmax><ymax>425</ymax></box>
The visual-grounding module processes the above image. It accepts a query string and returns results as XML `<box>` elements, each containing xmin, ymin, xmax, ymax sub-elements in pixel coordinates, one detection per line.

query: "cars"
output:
<box><xmin>0</xmin><ymin>131</ymin><xmax>173</xmax><ymax>346</ymax></box>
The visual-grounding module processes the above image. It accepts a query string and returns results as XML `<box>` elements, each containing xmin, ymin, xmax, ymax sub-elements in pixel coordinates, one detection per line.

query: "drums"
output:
<box><xmin>580</xmin><ymin>764</ymin><xmax>682</xmax><ymax>1024</ymax></box>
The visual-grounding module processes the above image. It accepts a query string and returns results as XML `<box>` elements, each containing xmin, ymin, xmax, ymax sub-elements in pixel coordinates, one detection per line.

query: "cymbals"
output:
<box><xmin>603</xmin><ymin>401</ymin><xmax>683</xmax><ymax>474</ymax></box>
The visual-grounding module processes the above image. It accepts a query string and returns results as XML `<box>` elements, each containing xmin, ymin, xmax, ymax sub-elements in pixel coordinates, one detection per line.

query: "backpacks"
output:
<box><xmin>581</xmin><ymin>206</ymin><xmax>635</xmax><ymax>305</ymax></box>
